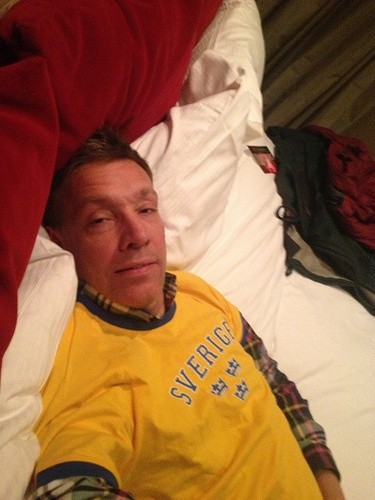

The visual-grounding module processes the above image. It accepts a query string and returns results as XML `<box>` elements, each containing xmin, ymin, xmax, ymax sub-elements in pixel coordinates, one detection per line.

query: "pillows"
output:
<box><xmin>130</xmin><ymin>69</ymin><xmax>248</xmax><ymax>269</ymax></box>
<box><xmin>0</xmin><ymin>236</ymin><xmax>78</xmax><ymax>500</ymax></box>
<box><xmin>177</xmin><ymin>2</ymin><xmax>266</xmax><ymax>115</ymax></box>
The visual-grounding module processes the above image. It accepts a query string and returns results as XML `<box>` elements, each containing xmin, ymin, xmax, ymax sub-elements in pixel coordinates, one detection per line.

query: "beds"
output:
<box><xmin>1</xmin><ymin>1</ymin><xmax>375</xmax><ymax>499</ymax></box>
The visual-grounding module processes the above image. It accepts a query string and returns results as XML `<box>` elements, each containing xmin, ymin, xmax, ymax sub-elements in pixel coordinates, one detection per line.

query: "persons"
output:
<box><xmin>24</xmin><ymin>137</ymin><xmax>346</xmax><ymax>499</ymax></box>
